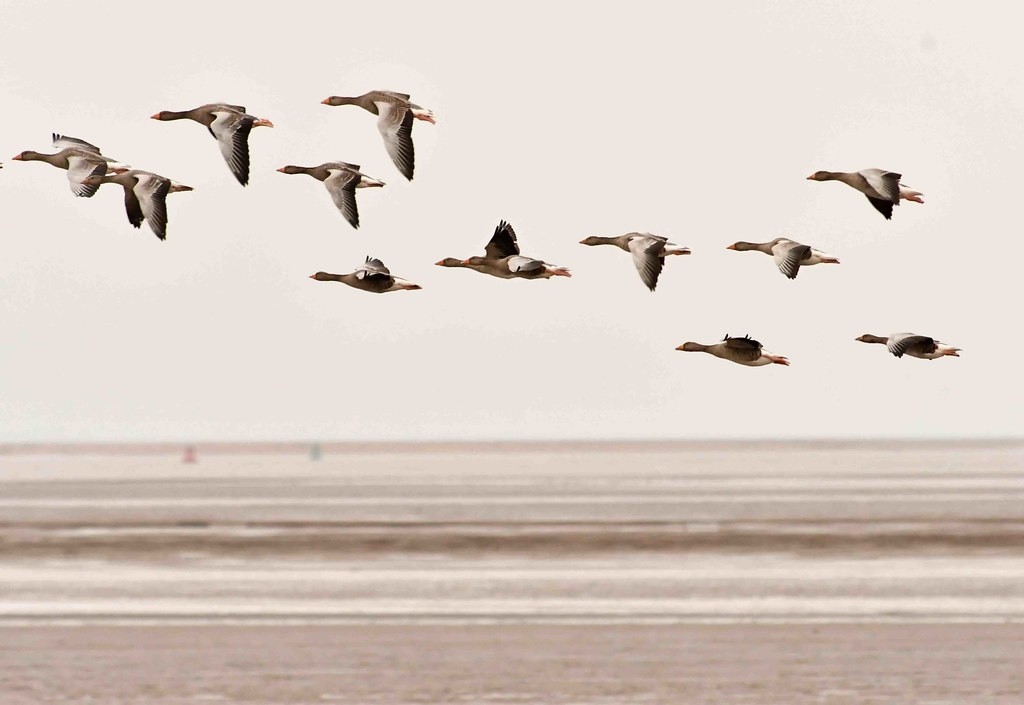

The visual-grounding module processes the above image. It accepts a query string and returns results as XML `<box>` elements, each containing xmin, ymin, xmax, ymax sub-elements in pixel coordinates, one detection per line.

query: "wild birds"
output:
<box><xmin>309</xmin><ymin>255</ymin><xmax>422</xmax><ymax>294</ymax></box>
<box><xmin>150</xmin><ymin>103</ymin><xmax>274</xmax><ymax>188</ymax></box>
<box><xmin>80</xmin><ymin>169</ymin><xmax>194</xmax><ymax>242</ymax></box>
<box><xmin>434</xmin><ymin>219</ymin><xmax>520</xmax><ymax>280</ymax></box>
<box><xmin>673</xmin><ymin>333</ymin><xmax>790</xmax><ymax>368</ymax></box>
<box><xmin>11</xmin><ymin>133</ymin><xmax>132</xmax><ymax>198</ymax></box>
<box><xmin>578</xmin><ymin>231</ymin><xmax>691</xmax><ymax>291</ymax></box>
<box><xmin>807</xmin><ymin>168</ymin><xmax>925</xmax><ymax>220</ymax></box>
<box><xmin>727</xmin><ymin>238</ymin><xmax>840</xmax><ymax>280</ymax></box>
<box><xmin>276</xmin><ymin>162</ymin><xmax>385</xmax><ymax>230</ymax></box>
<box><xmin>854</xmin><ymin>333</ymin><xmax>962</xmax><ymax>361</ymax></box>
<box><xmin>320</xmin><ymin>90</ymin><xmax>435</xmax><ymax>181</ymax></box>
<box><xmin>461</xmin><ymin>255</ymin><xmax>572</xmax><ymax>278</ymax></box>
